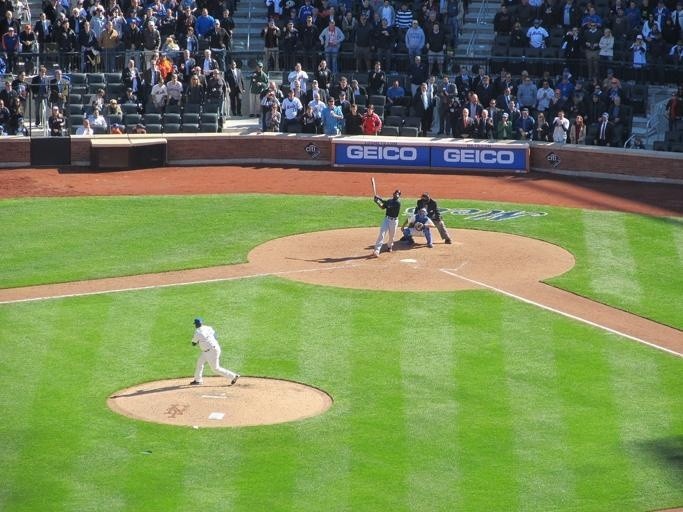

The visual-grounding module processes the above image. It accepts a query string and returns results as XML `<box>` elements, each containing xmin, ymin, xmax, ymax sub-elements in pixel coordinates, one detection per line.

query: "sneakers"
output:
<box><xmin>191</xmin><ymin>381</ymin><xmax>200</xmax><ymax>384</ymax></box>
<box><xmin>231</xmin><ymin>374</ymin><xmax>240</xmax><ymax>384</ymax></box>
<box><xmin>373</xmin><ymin>237</ymin><xmax>452</xmax><ymax>257</ymax></box>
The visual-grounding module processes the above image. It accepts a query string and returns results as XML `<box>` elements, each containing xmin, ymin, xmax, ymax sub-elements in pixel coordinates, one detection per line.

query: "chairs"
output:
<box><xmin>483</xmin><ymin>0</ymin><xmax>682</xmax><ymax>155</ymax></box>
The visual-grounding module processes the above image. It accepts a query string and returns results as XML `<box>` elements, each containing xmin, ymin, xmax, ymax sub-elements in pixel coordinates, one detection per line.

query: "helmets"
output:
<box><xmin>422</xmin><ymin>193</ymin><xmax>430</xmax><ymax>203</ymax></box>
<box><xmin>418</xmin><ymin>208</ymin><xmax>428</xmax><ymax>218</ymax></box>
<box><xmin>394</xmin><ymin>190</ymin><xmax>401</xmax><ymax>196</ymax></box>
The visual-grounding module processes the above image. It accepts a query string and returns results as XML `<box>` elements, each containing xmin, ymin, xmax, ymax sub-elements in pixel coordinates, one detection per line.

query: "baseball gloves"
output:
<box><xmin>415</xmin><ymin>221</ymin><xmax>424</xmax><ymax>232</ymax></box>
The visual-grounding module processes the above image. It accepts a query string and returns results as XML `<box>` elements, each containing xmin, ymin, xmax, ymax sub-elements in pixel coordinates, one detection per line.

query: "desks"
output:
<box><xmin>90</xmin><ymin>139</ymin><xmax>166</xmax><ymax>171</ymax></box>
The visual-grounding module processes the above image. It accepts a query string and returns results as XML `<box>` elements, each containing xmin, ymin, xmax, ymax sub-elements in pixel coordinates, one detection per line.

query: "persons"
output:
<box><xmin>413</xmin><ymin>191</ymin><xmax>453</xmax><ymax>244</ymax></box>
<box><xmin>373</xmin><ymin>188</ymin><xmax>402</xmax><ymax>258</ymax></box>
<box><xmin>189</xmin><ymin>317</ymin><xmax>241</xmax><ymax>389</ymax></box>
<box><xmin>0</xmin><ymin>1</ymin><xmax>683</xmax><ymax>142</ymax></box>
<box><xmin>401</xmin><ymin>207</ymin><xmax>436</xmax><ymax>249</ymax></box>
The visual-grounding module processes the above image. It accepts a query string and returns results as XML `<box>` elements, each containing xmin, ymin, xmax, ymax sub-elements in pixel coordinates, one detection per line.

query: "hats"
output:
<box><xmin>193</xmin><ymin>318</ymin><xmax>202</xmax><ymax>324</ymax></box>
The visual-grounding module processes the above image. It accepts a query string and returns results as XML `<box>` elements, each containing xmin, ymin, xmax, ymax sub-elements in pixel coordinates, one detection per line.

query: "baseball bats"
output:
<box><xmin>372</xmin><ymin>178</ymin><xmax>377</xmax><ymax>200</ymax></box>
